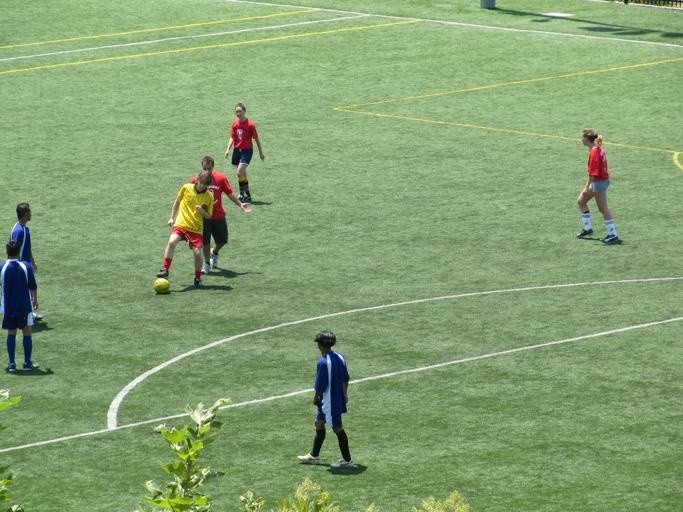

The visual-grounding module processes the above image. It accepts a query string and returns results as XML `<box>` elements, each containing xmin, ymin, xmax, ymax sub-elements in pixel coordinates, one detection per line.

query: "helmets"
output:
<box><xmin>312</xmin><ymin>330</ymin><xmax>336</xmax><ymax>348</ymax></box>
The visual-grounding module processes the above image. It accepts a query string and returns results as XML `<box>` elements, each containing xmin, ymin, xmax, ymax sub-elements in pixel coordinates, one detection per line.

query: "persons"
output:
<box><xmin>223</xmin><ymin>102</ymin><xmax>266</xmax><ymax>203</ymax></box>
<box><xmin>191</xmin><ymin>155</ymin><xmax>252</xmax><ymax>274</ymax></box>
<box><xmin>576</xmin><ymin>128</ymin><xmax>619</xmax><ymax>243</ymax></box>
<box><xmin>155</xmin><ymin>171</ymin><xmax>213</xmax><ymax>288</ymax></box>
<box><xmin>0</xmin><ymin>241</ymin><xmax>39</xmax><ymax>372</ymax></box>
<box><xmin>297</xmin><ymin>331</ymin><xmax>355</xmax><ymax>468</ymax></box>
<box><xmin>9</xmin><ymin>202</ymin><xmax>44</xmax><ymax>319</ymax></box>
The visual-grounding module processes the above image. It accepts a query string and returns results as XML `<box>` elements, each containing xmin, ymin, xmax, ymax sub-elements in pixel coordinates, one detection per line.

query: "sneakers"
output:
<box><xmin>156</xmin><ymin>268</ymin><xmax>168</xmax><ymax>278</ymax></box>
<box><xmin>238</xmin><ymin>195</ymin><xmax>244</xmax><ymax>202</ymax></box>
<box><xmin>211</xmin><ymin>247</ymin><xmax>218</xmax><ymax>268</ymax></box>
<box><xmin>23</xmin><ymin>362</ymin><xmax>40</xmax><ymax>370</ymax></box>
<box><xmin>576</xmin><ymin>228</ymin><xmax>593</xmax><ymax>238</ymax></box>
<box><xmin>200</xmin><ymin>262</ymin><xmax>210</xmax><ymax>274</ymax></box>
<box><xmin>242</xmin><ymin>197</ymin><xmax>251</xmax><ymax>202</ymax></box>
<box><xmin>9</xmin><ymin>363</ymin><xmax>16</xmax><ymax>372</ymax></box>
<box><xmin>194</xmin><ymin>277</ymin><xmax>201</xmax><ymax>289</ymax></box>
<box><xmin>329</xmin><ymin>458</ymin><xmax>353</xmax><ymax>468</ymax></box>
<box><xmin>600</xmin><ymin>235</ymin><xmax>618</xmax><ymax>243</ymax></box>
<box><xmin>32</xmin><ymin>312</ymin><xmax>43</xmax><ymax>320</ymax></box>
<box><xmin>298</xmin><ymin>452</ymin><xmax>319</xmax><ymax>464</ymax></box>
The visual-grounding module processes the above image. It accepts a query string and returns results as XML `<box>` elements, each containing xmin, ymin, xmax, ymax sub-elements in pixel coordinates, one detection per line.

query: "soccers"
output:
<box><xmin>153</xmin><ymin>278</ymin><xmax>169</xmax><ymax>294</ymax></box>
